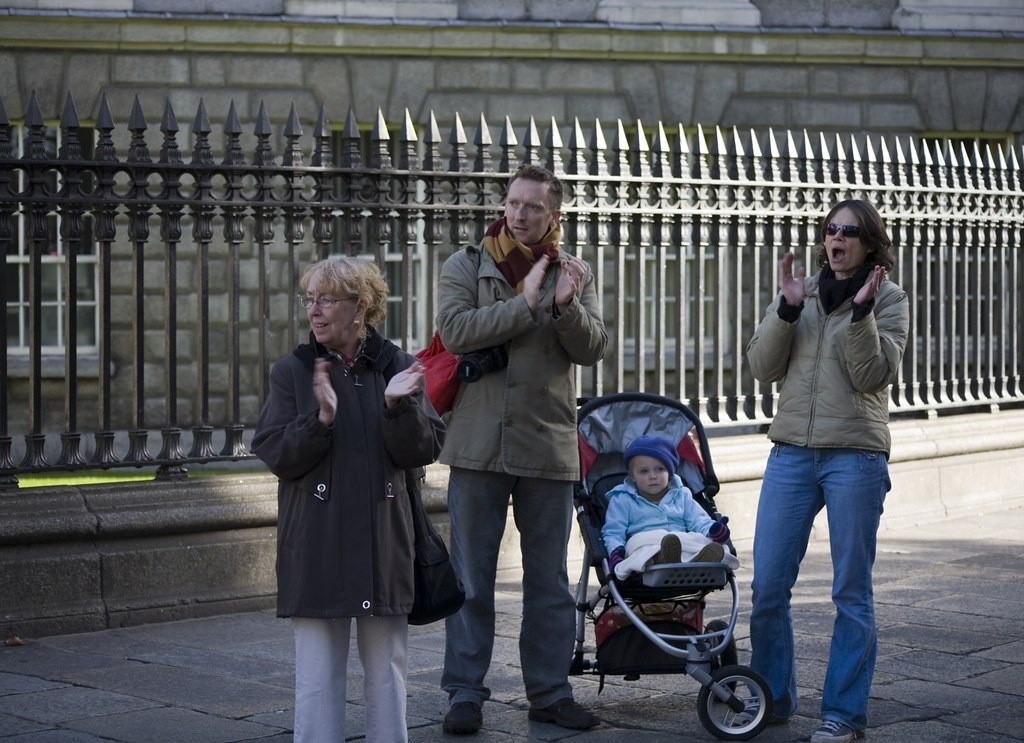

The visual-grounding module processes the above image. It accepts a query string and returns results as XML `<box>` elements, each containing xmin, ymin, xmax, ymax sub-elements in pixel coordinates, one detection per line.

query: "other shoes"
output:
<box><xmin>443</xmin><ymin>702</ymin><xmax>483</xmax><ymax>736</ymax></box>
<box><xmin>690</xmin><ymin>542</ymin><xmax>724</xmax><ymax>563</ymax></box>
<box><xmin>529</xmin><ymin>697</ymin><xmax>600</xmax><ymax>728</ymax></box>
<box><xmin>658</xmin><ymin>534</ymin><xmax>681</xmax><ymax>563</ymax></box>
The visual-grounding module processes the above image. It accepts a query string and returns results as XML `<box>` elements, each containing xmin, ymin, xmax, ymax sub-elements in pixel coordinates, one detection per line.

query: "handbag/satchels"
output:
<box><xmin>414</xmin><ymin>329</ymin><xmax>461</xmax><ymax>416</ymax></box>
<box><xmin>405</xmin><ymin>462</ymin><xmax>467</xmax><ymax>626</ymax></box>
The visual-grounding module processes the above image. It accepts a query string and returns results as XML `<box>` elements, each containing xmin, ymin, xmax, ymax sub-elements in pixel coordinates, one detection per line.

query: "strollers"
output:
<box><xmin>571</xmin><ymin>391</ymin><xmax>772</xmax><ymax>743</ymax></box>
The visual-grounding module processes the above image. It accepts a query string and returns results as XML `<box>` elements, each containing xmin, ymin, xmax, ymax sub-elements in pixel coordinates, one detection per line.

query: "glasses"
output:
<box><xmin>825</xmin><ymin>223</ymin><xmax>860</xmax><ymax>238</ymax></box>
<box><xmin>300</xmin><ymin>296</ymin><xmax>355</xmax><ymax>311</ymax></box>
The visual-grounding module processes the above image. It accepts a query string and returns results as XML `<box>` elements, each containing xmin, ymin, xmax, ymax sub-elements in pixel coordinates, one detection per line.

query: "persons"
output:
<box><xmin>601</xmin><ymin>433</ymin><xmax>740</xmax><ymax>581</ymax></box>
<box><xmin>435</xmin><ymin>165</ymin><xmax>610</xmax><ymax>733</ymax></box>
<box><xmin>729</xmin><ymin>199</ymin><xmax>910</xmax><ymax>743</ymax></box>
<box><xmin>250</xmin><ymin>257</ymin><xmax>447</xmax><ymax>743</ymax></box>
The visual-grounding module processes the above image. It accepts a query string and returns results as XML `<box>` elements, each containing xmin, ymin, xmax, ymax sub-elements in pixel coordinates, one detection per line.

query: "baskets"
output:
<box><xmin>643</xmin><ymin>562</ymin><xmax>728</xmax><ymax>586</ymax></box>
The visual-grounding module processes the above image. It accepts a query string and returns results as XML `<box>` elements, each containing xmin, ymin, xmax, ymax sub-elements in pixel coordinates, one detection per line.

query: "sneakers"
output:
<box><xmin>810</xmin><ymin>720</ymin><xmax>859</xmax><ymax>743</ymax></box>
<box><xmin>730</xmin><ymin>700</ymin><xmax>789</xmax><ymax>729</ymax></box>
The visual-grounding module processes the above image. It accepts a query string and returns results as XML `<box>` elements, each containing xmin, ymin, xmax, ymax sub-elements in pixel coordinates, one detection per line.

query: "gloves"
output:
<box><xmin>609</xmin><ymin>546</ymin><xmax>626</xmax><ymax>573</ymax></box>
<box><xmin>709</xmin><ymin>516</ymin><xmax>730</xmax><ymax>545</ymax></box>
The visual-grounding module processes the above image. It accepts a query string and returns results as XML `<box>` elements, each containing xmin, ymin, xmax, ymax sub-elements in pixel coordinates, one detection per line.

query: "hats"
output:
<box><xmin>624</xmin><ymin>434</ymin><xmax>679</xmax><ymax>477</ymax></box>
